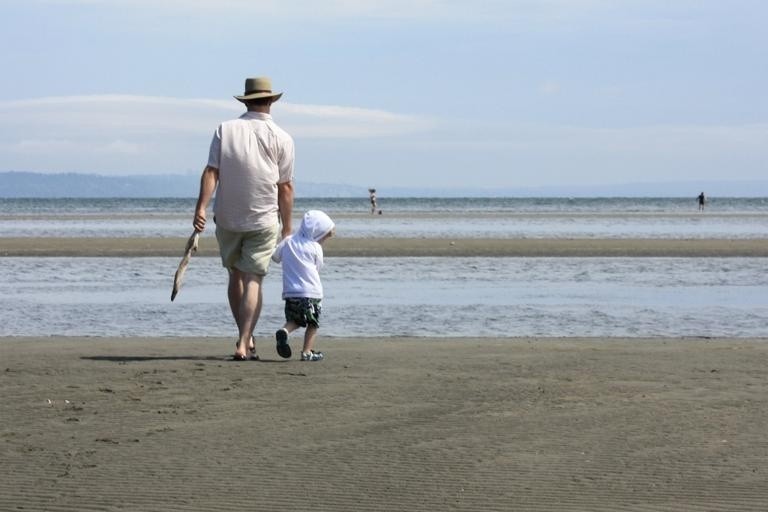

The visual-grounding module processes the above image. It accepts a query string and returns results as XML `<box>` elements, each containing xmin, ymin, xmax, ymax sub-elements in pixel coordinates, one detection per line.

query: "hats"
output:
<box><xmin>233</xmin><ymin>77</ymin><xmax>283</xmax><ymax>107</ymax></box>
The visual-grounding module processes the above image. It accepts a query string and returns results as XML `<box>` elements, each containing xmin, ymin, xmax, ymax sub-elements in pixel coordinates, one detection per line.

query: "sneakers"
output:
<box><xmin>302</xmin><ymin>349</ymin><xmax>324</xmax><ymax>361</ymax></box>
<box><xmin>275</xmin><ymin>328</ymin><xmax>292</xmax><ymax>359</ymax></box>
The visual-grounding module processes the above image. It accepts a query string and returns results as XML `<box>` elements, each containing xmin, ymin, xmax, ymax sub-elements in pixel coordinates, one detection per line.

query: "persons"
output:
<box><xmin>272</xmin><ymin>210</ymin><xmax>336</xmax><ymax>361</ymax></box>
<box><xmin>368</xmin><ymin>188</ymin><xmax>377</xmax><ymax>214</ymax></box>
<box><xmin>696</xmin><ymin>191</ymin><xmax>705</xmax><ymax>213</ymax></box>
<box><xmin>193</xmin><ymin>77</ymin><xmax>296</xmax><ymax>359</ymax></box>
